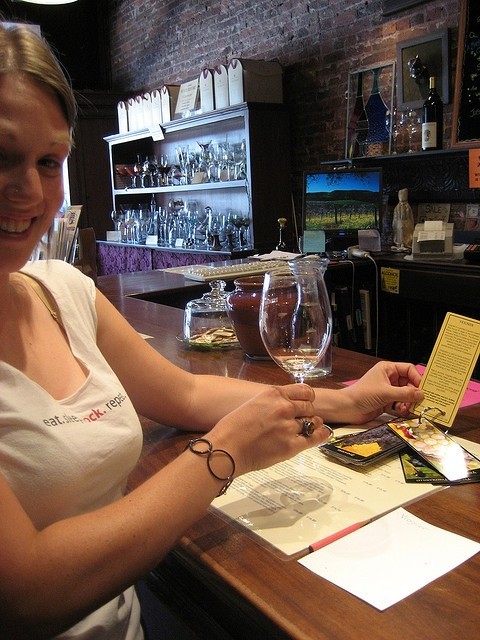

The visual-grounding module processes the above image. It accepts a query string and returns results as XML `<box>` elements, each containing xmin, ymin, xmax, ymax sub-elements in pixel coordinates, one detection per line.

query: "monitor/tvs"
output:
<box><xmin>301</xmin><ymin>167</ymin><xmax>384</xmax><ymax>245</ymax></box>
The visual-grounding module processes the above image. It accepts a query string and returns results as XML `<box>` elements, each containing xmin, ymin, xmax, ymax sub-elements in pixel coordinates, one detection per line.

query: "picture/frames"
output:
<box><xmin>344</xmin><ymin>61</ymin><xmax>395</xmax><ymax>158</ymax></box>
<box><xmin>395</xmin><ymin>27</ymin><xmax>452</xmax><ymax>112</ymax></box>
<box><xmin>449</xmin><ymin>0</ymin><xmax>480</xmax><ymax>150</ymax></box>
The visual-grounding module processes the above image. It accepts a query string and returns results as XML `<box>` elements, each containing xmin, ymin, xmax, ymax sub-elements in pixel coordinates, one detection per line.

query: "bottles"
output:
<box><xmin>285</xmin><ymin>256</ymin><xmax>330</xmax><ymax>378</ymax></box>
<box><xmin>276</xmin><ymin>218</ymin><xmax>289</xmax><ymax>253</ymax></box>
<box><xmin>422</xmin><ymin>76</ymin><xmax>444</xmax><ymax>151</ymax></box>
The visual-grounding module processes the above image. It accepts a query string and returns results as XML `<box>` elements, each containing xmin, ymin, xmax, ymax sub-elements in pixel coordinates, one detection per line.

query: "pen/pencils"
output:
<box><xmin>309</xmin><ymin>519</ymin><xmax>372</xmax><ymax>553</ymax></box>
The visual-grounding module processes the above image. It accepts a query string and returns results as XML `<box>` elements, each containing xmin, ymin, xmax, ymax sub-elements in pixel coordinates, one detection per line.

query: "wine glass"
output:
<box><xmin>115</xmin><ymin>139</ymin><xmax>246</xmax><ymax>190</ymax></box>
<box><xmin>384</xmin><ymin>110</ymin><xmax>404</xmax><ymax>152</ymax></box>
<box><xmin>110</xmin><ymin>197</ymin><xmax>249</xmax><ymax>252</ymax></box>
<box><xmin>401</xmin><ymin>106</ymin><xmax>422</xmax><ymax>154</ymax></box>
<box><xmin>257</xmin><ymin>267</ymin><xmax>334</xmax><ymax>444</ymax></box>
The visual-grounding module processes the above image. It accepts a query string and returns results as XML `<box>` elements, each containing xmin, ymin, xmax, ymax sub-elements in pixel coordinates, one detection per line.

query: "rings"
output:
<box><xmin>294</xmin><ymin>414</ymin><xmax>316</xmax><ymax>439</ymax></box>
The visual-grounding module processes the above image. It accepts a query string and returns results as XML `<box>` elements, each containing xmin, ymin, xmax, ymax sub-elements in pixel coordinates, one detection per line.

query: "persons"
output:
<box><xmin>0</xmin><ymin>19</ymin><xmax>423</xmax><ymax>640</ymax></box>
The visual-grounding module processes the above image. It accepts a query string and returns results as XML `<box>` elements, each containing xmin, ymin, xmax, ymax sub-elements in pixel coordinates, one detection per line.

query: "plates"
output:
<box><xmin>176</xmin><ymin>330</ymin><xmax>241</xmax><ymax>351</ymax></box>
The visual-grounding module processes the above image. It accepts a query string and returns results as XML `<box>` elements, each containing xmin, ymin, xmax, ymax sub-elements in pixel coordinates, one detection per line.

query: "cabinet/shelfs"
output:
<box><xmin>379</xmin><ymin>261</ymin><xmax>478</xmax><ymax>382</ymax></box>
<box><xmin>103</xmin><ymin>101</ymin><xmax>289</xmax><ymax>275</ymax></box>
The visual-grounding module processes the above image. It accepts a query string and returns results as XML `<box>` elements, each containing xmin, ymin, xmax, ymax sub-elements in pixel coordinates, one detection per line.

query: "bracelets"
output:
<box><xmin>183</xmin><ymin>435</ymin><xmax>241</xmax><ymax>498</ymax></box>
